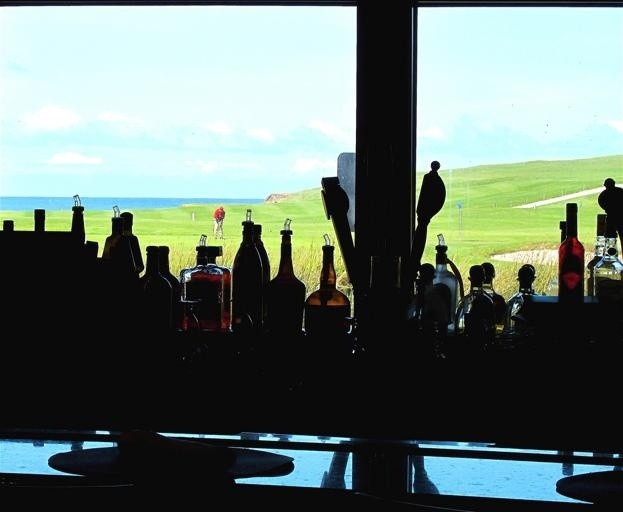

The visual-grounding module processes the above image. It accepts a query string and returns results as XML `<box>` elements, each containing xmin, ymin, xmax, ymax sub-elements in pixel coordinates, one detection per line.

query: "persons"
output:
<box><xmin>210</xmin><ymin>203</ymin><xmax>226</xmax><ymax>238</ymax></box>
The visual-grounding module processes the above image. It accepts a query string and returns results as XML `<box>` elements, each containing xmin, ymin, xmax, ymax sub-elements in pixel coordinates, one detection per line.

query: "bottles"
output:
<box><xmin>587</xmin><ymin>215</ymin><xmax>623</xmax><ymax>300</ymax></box>
<box><xmin>267</xmin><ymin>229</ymin><xmax>306</xmax><ymax>335</ymax></box>
<box><xmin>414</xmin><ymin>246</ymin><xmax>509</xmax><ymax>347</ymax></box>
<box><xmin>102</xmin><ymin>212</ymin><xmax>270</xmax><ymax>335</ymax></box>
<box><xmin>506</xmin><ymin>265</ymin><xmax>535</xmax><ymax>337</ymax></box>
<box><xmin>559</xmin><ymin>203</ymin><xmax>584</xmax><ymax>304</ymax></box>
<box><xmin>305</xmin><ymin>245</ymin><xmax>350</xmax><ymax>339</ymax></box>
